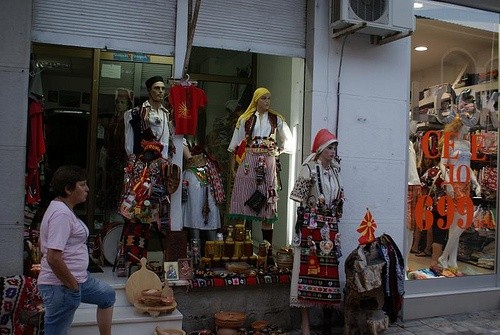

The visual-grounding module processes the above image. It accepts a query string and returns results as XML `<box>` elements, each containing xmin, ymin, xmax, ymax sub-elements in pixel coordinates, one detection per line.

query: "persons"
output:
<box><xmin>438</xmin><ymin>118</ymin><xmax>481</xmax><ymax>271</ymax></box>
<box><xmin>406</xmin><ymin>139</ymin><xmax>420</xmax><ymax>281</ymax></box>
<box><xmin>98</xmin><ymin>88</ymin><xmax>132</xmax><ymax>209</ymax></box>
<box><xmin>118</xmin><ymin>140</ymin><xmax>180</xmax><ymax>272</ymax></box>
<box><xmin>180</xmin><ymin>150</ymin><xmax>228</xmax><ymax>256</ymax></box>
<box><xmin>34</xmin><ymin>165</ymin><xmax>116</xmax><ymax>335</ymax></box>
<box><xmin>227</xmin><ymin>87</ymin><xmax>293</xmax><ymax>267</ymax></box>
<box><xmin>411</xmin><ymin>150</ymin><xmax>440</xmax><ymax>257</ymax></box>
<box><xmin>122</xmin><ymin>76</ymin><xmax>181</xmax><ymax>162</ymax></box>
<box><xmin>289</xmin><ymin>129</ymin><xmax>345</xmax><ymax>335</ymax></box>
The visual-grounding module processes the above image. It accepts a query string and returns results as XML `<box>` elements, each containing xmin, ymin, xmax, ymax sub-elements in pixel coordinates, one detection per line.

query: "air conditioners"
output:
<box><xmin>330</xmin><ymin>0</ymin><xmax>416</xmax><ymax>38</ymax></box>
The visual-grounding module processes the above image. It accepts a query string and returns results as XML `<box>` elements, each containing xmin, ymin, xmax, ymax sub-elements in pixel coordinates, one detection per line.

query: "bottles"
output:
<box><xmin>199</xmin><ymin>241</ymin><xmax>267</xmax><ymax>271</ymax></box>
<box><xmin>234</xmin><ymin>224</ymin><xmax>245</xmax><ymax>242</ymax></box>
<box><xmin>246</xmin><ymin>230</ymin><xmax>252</xmax><ymax>241</ymax></box>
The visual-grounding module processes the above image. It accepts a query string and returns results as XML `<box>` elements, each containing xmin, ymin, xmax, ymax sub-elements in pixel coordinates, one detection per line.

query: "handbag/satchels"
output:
<box><xmin>244</xmin><ymin>189</ymin><xmax>266</xmax><ymax>215</ymax></box>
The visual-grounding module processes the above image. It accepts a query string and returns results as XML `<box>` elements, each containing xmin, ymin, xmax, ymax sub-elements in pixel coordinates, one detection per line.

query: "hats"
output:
<box><xmin>145</xmin><ymin>76</ymin><xmax>164</xmax><ymax>88</ymax></box>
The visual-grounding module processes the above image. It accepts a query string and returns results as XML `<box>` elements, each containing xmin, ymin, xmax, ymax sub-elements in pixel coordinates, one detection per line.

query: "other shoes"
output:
<box><xmin>430</xmin><ymin>266</ymin><xmax>464</xmax><ymax>277</ymax></box>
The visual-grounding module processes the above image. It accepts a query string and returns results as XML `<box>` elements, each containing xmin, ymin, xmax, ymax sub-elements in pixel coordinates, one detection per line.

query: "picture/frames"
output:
<box><xmin>163</xmin><ymin>262</ymin><xmax>179</xmax><ymax>281</ymax></box>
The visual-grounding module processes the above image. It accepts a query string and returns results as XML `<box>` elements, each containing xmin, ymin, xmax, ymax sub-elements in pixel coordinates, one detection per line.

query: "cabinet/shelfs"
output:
<box><xmin>417</xmin><ymin>80</ymin><xmax>499</xmax><ymax>270</ymax></box>
